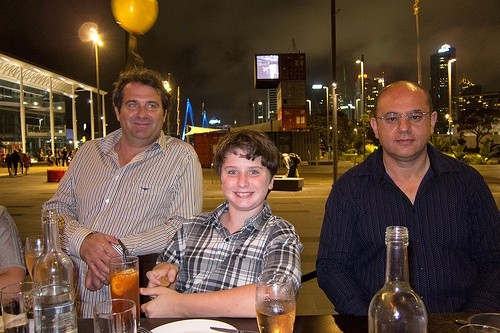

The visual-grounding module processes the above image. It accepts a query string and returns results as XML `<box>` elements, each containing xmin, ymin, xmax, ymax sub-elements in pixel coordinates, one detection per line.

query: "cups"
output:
<box><xmin>26</xmin><ymin>234</ymin><xmax>44</xmax><ymax>279</ymax></box>
<box><xmin>0</xmin><ymin>282</ymin><xmax>42</xmax><ymax>333</ymax></box>
<box><xmin>457</xmin><ymin>313</ymin><xmax>500</xmax><ymax>333</ymax></box>
<box><xmin>256</xmin><ymin>272</ymin><xmax>296</xmax><ymax>333</ymax></box>
<box><xmin>108</xmin><ymin>256</ymin><xmax>140</xmax><ymax>328</ymax></box>
<box><xmin>93</xmin><ymin>298</ymin><xmax>137</xmax><ymax>333</ymax></box>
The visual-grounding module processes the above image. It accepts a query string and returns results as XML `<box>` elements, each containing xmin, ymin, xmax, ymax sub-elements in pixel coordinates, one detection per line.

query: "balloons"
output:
<box><xmin>112</xmin><ymin>0</ymin><xmax>158</xmax><ymax>34</ymax></box>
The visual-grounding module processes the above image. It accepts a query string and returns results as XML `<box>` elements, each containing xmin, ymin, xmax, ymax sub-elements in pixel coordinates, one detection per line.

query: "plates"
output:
<box><xmin>151</xmin><ymin>320</ymin><xmax>238</xmax><ymax>333</ymax></box>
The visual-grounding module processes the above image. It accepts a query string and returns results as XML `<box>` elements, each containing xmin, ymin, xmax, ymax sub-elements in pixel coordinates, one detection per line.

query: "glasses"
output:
<box><xmin>376</xmin><ymin>111</ymin><xmax>430</xmax><ymax>127</ymax></box>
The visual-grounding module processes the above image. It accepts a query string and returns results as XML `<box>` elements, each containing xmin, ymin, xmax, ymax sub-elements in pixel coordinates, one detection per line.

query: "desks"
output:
<box><xmin>0</xmin><ymin>308</ymin><xmax>500</xmax><ymax>333</ymax></box>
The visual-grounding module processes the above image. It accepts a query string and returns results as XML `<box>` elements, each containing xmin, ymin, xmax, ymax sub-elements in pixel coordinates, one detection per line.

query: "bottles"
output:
<box><xmin>32</xmin><ymin>209</ymin><xmax>79</xmax><ymax>333</ymax></box>
<box><xmin>367</xmin><ymin>226</ymin><xmax>428</xmax><ymax>333</ymax></box>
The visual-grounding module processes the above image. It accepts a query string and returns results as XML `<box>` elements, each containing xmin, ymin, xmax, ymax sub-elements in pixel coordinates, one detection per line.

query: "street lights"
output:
<box><xmin>311</xmin><ymin>84</ymin><xmax>329</xmax><ymax>145</ymax></box>
<box><xmin>447</xmin><ymin>59</ymin><xmax>457</xmax><ymax>116</ymax></box>
<box><xmin>89</xmin><ymin>28</ymin><xmax>103</xmax><ymax>141</ymax></box>
<box><xmin>355</xmin><ymin>59</ymin><xmax>364</xmax><ymax>115</ymax></box>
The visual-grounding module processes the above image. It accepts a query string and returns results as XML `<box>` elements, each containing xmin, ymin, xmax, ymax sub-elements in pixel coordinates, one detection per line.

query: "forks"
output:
<box><xmin>137</xmin><ymin>326</ymin><xmax>152</xmax><ymax>333</ymax></box>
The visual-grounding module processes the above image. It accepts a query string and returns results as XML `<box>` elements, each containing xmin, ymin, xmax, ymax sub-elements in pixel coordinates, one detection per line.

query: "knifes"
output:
<box><xmin>210</xmin><ymin>327</ymin><xmax>258</xmax><ymax>333</ymax></box>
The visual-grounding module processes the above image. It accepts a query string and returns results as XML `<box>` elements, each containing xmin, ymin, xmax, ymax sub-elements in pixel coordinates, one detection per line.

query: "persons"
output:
<box><xmin>40</xmin><ymin>146</ymin><xmax>78</xmax><ymax>166</ymax></box>
<box><xmin>313</xmin><ymin>79</ymin><xmax>500</xmax><ymax>317</ymax></box>
<box><xmin>40</xmin><ymin>67</ymin><xmax>204</xmax><ymax>333</ymax></box>
<box><xmin>138</xmin><ymin>128</ymin><xmax>304</xmax><ymax>318</ymax></box>
<box><xmin>5</xmin><ymin>148</ymin><xmax>32</xmax><ymax>177</ymax></box>
<box><xmin>280</xmin><ymin>152</ymin><xmax>299</xmax><ymax>177</ymax></box>
<box><xmin>0</xmin><ymin>204</ymin><xmax>27</xmax><ymax>312</ymax></box>
